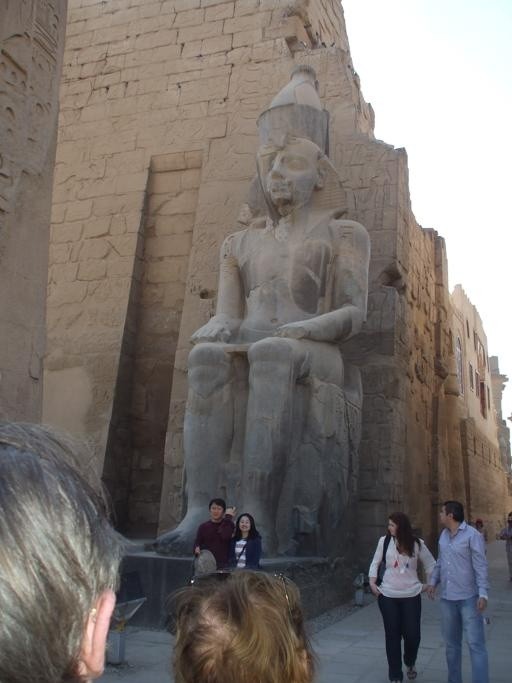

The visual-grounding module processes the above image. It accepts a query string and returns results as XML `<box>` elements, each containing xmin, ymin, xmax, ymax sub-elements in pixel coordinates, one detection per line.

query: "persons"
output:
<box><xmin>423</xmin><ymin>498</ymin><xmax>490</xmax><ymax>683</ymax></box>
<box><xmin>140</xmin><ymin>70</ymin><xmax>373</xmax><ymax>565</ymax></box>
<box><xmin>184</xmin><ymin>498</ymin><xmax>237</xmax><ymax>584</ymax></box>
<box><xmin>496</xmin><ymin>512</ymin><xmax>511</xmax><ymax>583</ymax></box>
<box><xmin>475</xmin><ymin>515</ymin><xmax>488</xmax><ymax>556</ymax></box>
<box><xmin>167</xmin><ymin>564</ymin><xmax>317</xmax><ymax>682</ymax></box>
<box><xmin>1</xmin><ymin>419</ymin><xmax>131</xmax><ymax>683</ymax></box>
<box><xmin>226</xmin><ymin>513</ymin><xmax>263</xmax><ymax>573</ymax></box>
<box><xmin>368</xmin><ymin>511</ymin><xmax>438</xmax><ymax>683</ymax></box>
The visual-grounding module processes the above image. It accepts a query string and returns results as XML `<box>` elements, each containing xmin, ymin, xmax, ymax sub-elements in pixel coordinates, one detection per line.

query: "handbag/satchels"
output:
<box><xmin>228</xmin><ymin>544</ymin><xmax>247</xmax><ymax>572</ymax></box>
<box><xmin>374</xmin><ymin>534</ymin><xmax>391</xmax><ymax>587</ymax></box>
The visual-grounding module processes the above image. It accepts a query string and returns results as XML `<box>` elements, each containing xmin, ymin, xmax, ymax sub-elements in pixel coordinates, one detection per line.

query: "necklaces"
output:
<box><xmin>392</xmin><ymin>540</ymin><xmax>410</xmax><ymax>575</ymax></box>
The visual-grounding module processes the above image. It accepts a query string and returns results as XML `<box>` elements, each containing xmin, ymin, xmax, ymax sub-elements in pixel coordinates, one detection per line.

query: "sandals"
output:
<box><xmin>390</xmin><ymin>665</ymin><xmax>418</xmax><ymax>683</ymax></box>
